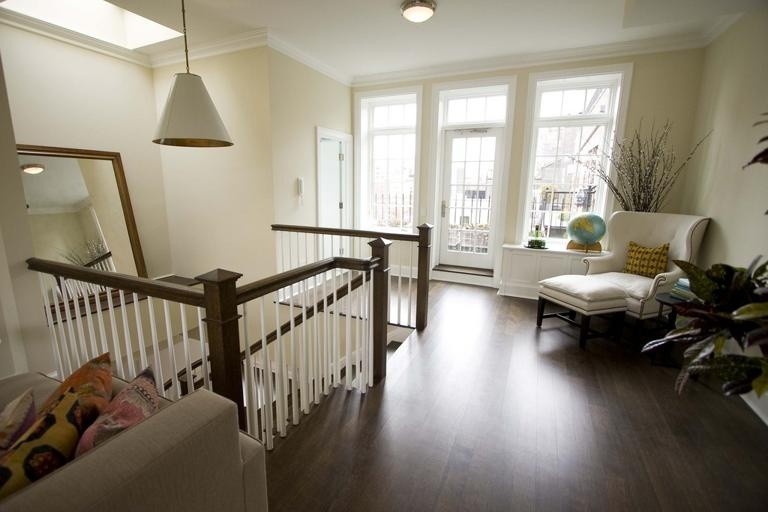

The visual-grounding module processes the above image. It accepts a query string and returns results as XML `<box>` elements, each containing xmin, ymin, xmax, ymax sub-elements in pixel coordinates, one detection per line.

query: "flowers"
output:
<box><xmin>576</xmin><ymin>117</ymin><xmax>716</xmax><ymax>211</ymax></box>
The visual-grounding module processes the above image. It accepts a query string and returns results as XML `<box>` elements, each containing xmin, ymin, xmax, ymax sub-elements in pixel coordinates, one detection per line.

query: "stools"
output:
<box><xmin>536</xmin><ymin>277</ymin><xmax>632</xmax><ymax>352</ymax></box>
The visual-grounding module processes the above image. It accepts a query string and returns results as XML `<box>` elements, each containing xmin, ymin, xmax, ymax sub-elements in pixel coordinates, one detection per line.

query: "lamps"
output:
<box><xmin>153</xmin><ymin>1</ymin><xmax>234</xmax><ymax>150</ymax></box>
<box><xmin>22</xmin><ymin>164</ymin><xmax>46</xmax><ymax>175</ymax></box>
<box><xmin>400</xmin><ymin>1</ymin><xmax>437</xmax><ymax>22</ymax></box>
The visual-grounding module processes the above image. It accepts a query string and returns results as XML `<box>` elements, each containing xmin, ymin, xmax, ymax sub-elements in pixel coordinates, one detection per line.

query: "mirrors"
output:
<box><xmin>14</xmin><ymin>142</ymin><xmax>150</xmax><ymax>325</ymax></box>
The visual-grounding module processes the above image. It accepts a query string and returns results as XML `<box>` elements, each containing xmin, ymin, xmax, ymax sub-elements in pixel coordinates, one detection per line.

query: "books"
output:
<box><xmin>669</xmin><ymin>278</ymin><xmax>706</xmax><ymax>304</ymax></box>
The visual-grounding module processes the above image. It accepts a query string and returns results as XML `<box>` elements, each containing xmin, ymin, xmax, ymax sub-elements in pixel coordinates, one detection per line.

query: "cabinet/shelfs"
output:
<box><xmin>496</xmin><ymin>243</ymin><xmax>608</xmax><ymax>303</ymax></box>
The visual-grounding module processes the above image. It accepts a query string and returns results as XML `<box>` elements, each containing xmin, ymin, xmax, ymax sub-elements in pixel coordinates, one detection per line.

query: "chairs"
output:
<box><xmin>0</xmin><ymin>362</ymin><xmax>270</xmax><ymax>512</ymax></box>
<box><xmin>449</xmin><ymin>228</ymin><xmax>488</xmax><ymax>253</ymax></box>
<box><xmin>581</xmin><ymin>210</ymin><xmax>712</xmax><ymax>361</ymax></box>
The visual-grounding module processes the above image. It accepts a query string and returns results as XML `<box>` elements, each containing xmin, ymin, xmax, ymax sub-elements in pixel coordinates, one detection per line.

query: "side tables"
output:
<box><xmin>649</xmin><ymin>292</ymin><xmax>719</xmax><ymax>382</ymax></box>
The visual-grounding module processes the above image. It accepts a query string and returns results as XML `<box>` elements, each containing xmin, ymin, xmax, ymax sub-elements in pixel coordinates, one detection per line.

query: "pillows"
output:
<box><xmin>623</xmin><ymin>239</ymin><xmax>670</xmax><ymax>279</ymax></box>
<box><xmin>537</xmin><ymin>274</ymin><xmax>630</xmax><ymax>302</ymax></box>
<box><xmin>0</xmin><ymin>353</ymin><xmax>159</xmax><ymax>496</ymax></box>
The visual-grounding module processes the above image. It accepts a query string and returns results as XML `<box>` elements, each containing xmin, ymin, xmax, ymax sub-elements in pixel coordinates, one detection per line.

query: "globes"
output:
<box><xmin>567</xmin><ymin>213</ymin><xmax>607</xmax><ymax>253</ymax></box>
